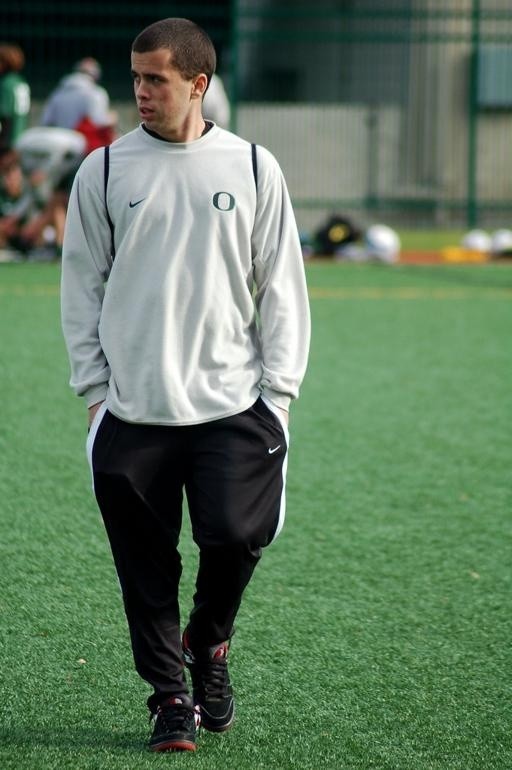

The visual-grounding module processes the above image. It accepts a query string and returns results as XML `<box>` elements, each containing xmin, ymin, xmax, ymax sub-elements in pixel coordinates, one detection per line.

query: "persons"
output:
<box><xmin>3</xmin><ymin>42</ymin><xmax>229</xmax><ymax>263</ymax></box>
<box><xmin>62</xmin><ymin>18</ymin><xmax>312</xmax><ymax>753</ymax></box>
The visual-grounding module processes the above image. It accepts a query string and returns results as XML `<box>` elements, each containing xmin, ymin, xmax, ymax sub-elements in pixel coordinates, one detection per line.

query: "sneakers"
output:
<box><xmin>144</xmin><ymin>693</ymin><xmax>200</xmax><ymax>753</ymax></box>
<box><xmin>181</xmin><ymin>624</ymin><xmax>235</xmax><ymax>733</ymax></box>
